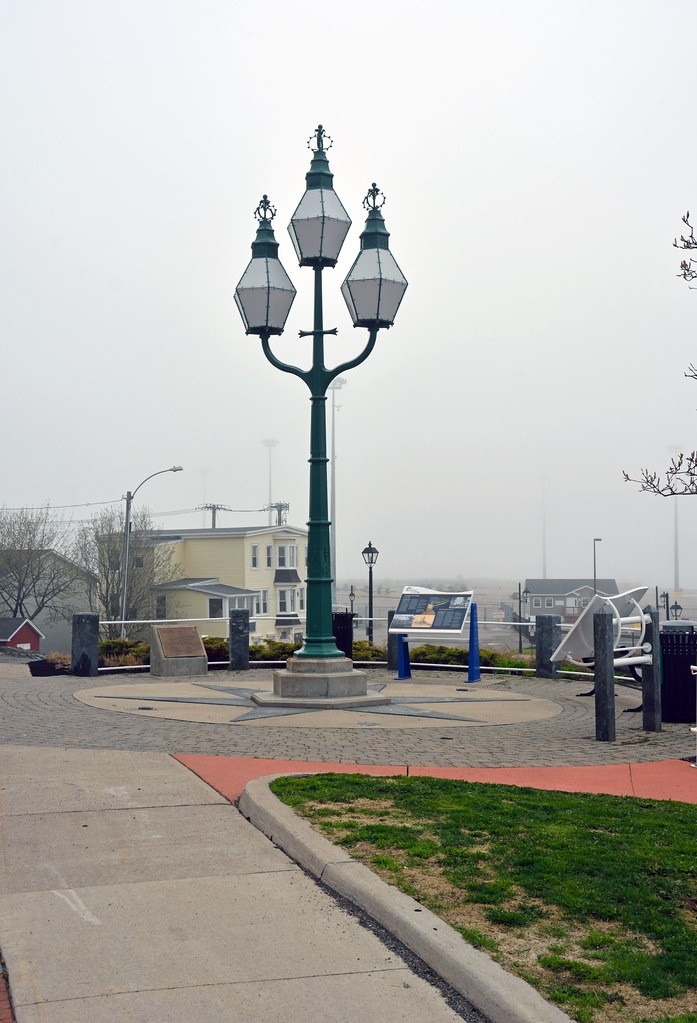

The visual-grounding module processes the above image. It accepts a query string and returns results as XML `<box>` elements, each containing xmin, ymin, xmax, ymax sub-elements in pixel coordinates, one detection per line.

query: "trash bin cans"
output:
<box><xmin>333</xmin><ymin>611</ymin><xmax>356</xmax><ymax>658</ymax></box>
<box><xmin>660</xmin><ymin>630</ymin><xmax>697</xmax><ymax>723</ymax></box>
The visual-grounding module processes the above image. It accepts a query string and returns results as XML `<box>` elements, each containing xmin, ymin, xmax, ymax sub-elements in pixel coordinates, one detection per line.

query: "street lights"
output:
<box><xmin>121</xmin><ymin>465</ymin><xmax>183</xmax><ymax>621</ymax></box>
<box><xmin>233</xmin><ymin>125</ymin><xmax>409</xmax><ymax>658</ymax></box>
<box><xmin>519</xmin><ymin>588</ymin><xmax>531</xmax><ymax>652</ymax></box>
<box><xmin>666</xmin><ymin>600</ymin><xmax>683</xmax><ymax>620</ymax></box>
<box><xmin>361</xmin><ymin>540</ymin><xmax>379</xmax><ymax>646</ymax></box>
<box><xmin>594</xmin><ymin>538</ymin><xmax>602</xmax><ymax>594</ymax></box>
<box><xmin>349</xmin><ymin>585</ymin><xmax>355</xmax><ymax>613</ymax></box>
